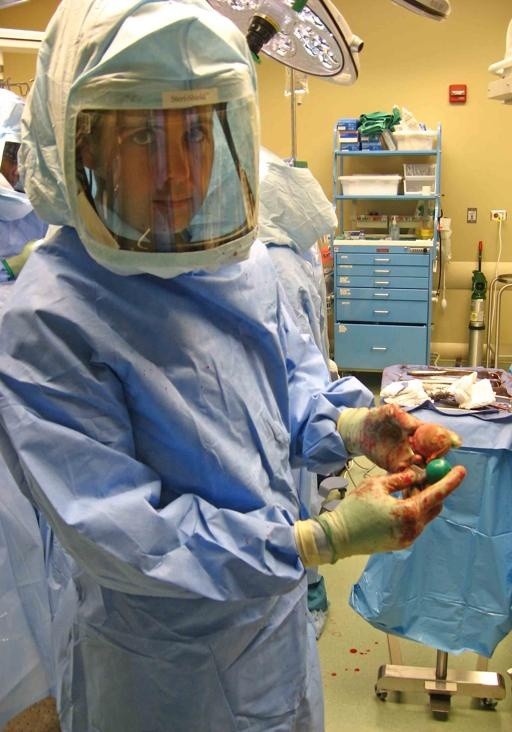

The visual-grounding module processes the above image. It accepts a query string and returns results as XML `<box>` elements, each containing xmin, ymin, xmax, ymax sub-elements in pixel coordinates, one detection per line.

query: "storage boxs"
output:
<box><xmin>339</xmin><ymin>129</ymin><xmax>359</xmax><ymax>142</ymax></box>
<box><xmin>362</xmin><ymin>143</ymin><xmax>381</xmax><ymax>152</ymax></box>
<box><xmin>338</xmin><ymin>118</ymin><xmax>359</xmax><ymax>131</ymax></box>
<box><xmin>337</xmin><ymin>174</ymin><xmax>402</xmax><ymax>197</ymax></box>
<box><xmin>394</xmin><ymin>129</ymin><xmax>435</xmax><ymax>152</ymax></box>
<box><xmin>403</xmin><ymin>163</ymin><xmax>437</xmax><ymax>194</ymax></box>
<box><xmin>360</xmin><ymin>133</ymin><xmax>380</xmax><ymax>143</ymax></box>
<box><xmin>340</xmin><ymin>143</ymin><xmax>359</xmax><ymax>151</ymax></box>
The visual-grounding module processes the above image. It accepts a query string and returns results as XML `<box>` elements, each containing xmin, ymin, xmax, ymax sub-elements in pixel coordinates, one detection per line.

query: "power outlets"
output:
<box><xmin>467</xmin><ymin>207</ymin><xmax>477</xmax><ymax>223</ymax></box>
<box><xmin>490</xmin><ymin>211</ymin><xmax>506</xmax><ymax>222</ymax></box>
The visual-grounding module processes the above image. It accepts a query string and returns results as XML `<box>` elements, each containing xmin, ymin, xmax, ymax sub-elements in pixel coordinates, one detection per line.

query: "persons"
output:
<box><xmin>1</xmin><ymin>86</ymin><xmax>50</xmax><ymax>284</ymax></box>
<box><xmin>0</xmin><ymin>0</ymin><xmax>468</xmax><ymax>732</ymax></box>
<box><xmin>257</xmin><ymin>146</ymin><xmax>337</xmax><ymax>640</ymax></box>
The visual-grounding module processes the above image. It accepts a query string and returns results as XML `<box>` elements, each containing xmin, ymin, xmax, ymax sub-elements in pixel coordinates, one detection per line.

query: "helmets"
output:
<box><xmin>17</xmin><ymin>0</ymin><xmax>262</xmax><ymax>280</ymax></box>
<box><xmin>0</xmin><ymin>88</ymin><xmax>34</xmax><ymax>223</ymax></box>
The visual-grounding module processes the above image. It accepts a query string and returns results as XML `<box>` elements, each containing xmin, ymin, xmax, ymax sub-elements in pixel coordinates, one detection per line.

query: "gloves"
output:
<box><xmin>336</xmin><ymin>404</ymin><xmax>460</xmax><ymax>475</ymax></box>
<box><xmin>293</xmin><ymin>467</ymin><xmax>466</xmax><ymax>566</ymax></box>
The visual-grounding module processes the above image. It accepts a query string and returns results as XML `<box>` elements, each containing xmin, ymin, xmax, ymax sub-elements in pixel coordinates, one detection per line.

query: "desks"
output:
<box><xmin>351</xmin><ymin>366</ymin><xmax>511</xmax><ymax>720</ymax></box>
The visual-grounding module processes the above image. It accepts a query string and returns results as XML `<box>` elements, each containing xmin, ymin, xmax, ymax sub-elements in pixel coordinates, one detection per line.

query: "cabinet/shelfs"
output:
<box><xmin>329</xmin><ymin>121</ymin><xmax>440</xmax><ymax>374</ymax></box>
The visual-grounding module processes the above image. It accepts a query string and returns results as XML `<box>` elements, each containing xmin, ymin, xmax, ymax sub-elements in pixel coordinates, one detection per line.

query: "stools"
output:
<box><xmin>486</xmin><ymin>273</ymin><xmax>512</xmax><ymax>369</ymax></box>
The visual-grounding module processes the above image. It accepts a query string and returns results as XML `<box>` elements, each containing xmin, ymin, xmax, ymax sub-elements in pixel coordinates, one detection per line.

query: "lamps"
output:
<box><xmin>220</xmin><ymin>1</ymin><xmax>366</xmax><ymax>86</ymax></box>
